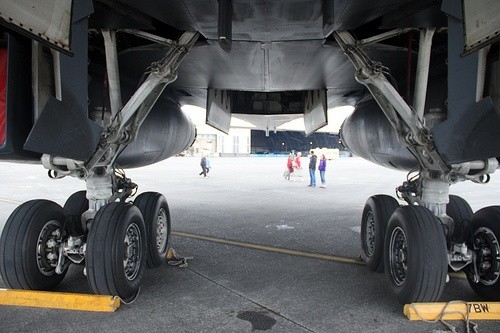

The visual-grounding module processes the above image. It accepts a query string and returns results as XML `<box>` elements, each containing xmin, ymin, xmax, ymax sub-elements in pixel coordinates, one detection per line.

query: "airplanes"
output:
<box><xmin>0</xmin><ymin>1</ymin><xmax>500</xmax><ymax>305</ymax></box>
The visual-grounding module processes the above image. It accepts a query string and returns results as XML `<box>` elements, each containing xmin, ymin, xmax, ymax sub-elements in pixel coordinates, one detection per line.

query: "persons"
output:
<box><xmin>308</xmin><ymin>151</ymin><xmax>317</xmax><ymax>187</ymax></box>
<box><xmin>318</xmin><ymin>153</ymin><xmax>327</xmax><ymax>188</ymax></box>
<box><xmin>198</xmin><ymin>153</ymin><xmax>212</xmax><ymax>178</ymax></box>
<box><xmin>283</xmin><ymin>149</ymin><xmax>303</xmax><ymax>181</ymax></box>
